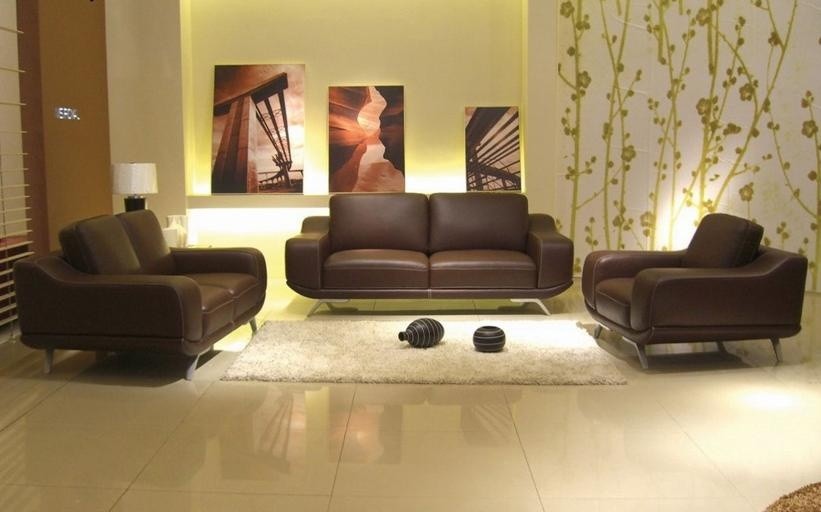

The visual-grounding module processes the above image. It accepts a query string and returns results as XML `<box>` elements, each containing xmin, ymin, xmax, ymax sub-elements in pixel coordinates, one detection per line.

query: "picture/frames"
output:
<box><xmin>211</xmin><ymin>64</ymin><xmax>306</xmax><ymax>194</ymax></box>
<box><xmin>328</xmin><ymin>86</ymin><xmax>405</xmax><ymax>193</ymax></box>
<box><xmin>464</xmin><ymin>106</ymin><xmax>522</xmax><ymax>192</ymax></box>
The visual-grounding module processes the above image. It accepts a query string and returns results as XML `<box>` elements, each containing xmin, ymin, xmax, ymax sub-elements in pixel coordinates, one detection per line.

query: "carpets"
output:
<box><xmin>222</xmin><ymin>319</ymin><xmax>626</xmax><ymax>383</ymax></box>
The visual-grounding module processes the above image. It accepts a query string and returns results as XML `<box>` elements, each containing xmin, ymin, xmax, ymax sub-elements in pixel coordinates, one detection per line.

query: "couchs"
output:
<box><xmin>284</xmin><ymin>190</ymin><xmax>574</xmax><ymax>315</ymax></box>
<box><xmin>13</xmin><ymin>209</ymin><xmax>267</xmax><ymax>381</ymax></box>
<box><xmin>581</xmin><ymin>214</ymin><xmax>808</xmax><ymax>369</ymax></box>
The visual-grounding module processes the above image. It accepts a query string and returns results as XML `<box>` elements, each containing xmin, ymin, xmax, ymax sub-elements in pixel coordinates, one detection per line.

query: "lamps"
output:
<box><xmin>112</xmin><ymin>163</ymin><xmax>159</xmax><ymax>213</ymax></box>
